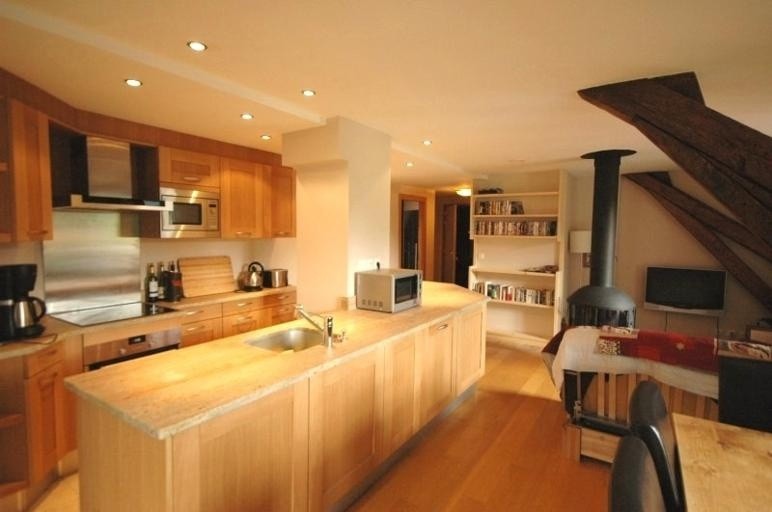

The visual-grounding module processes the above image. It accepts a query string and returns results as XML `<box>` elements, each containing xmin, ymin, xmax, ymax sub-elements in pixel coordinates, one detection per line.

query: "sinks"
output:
<box><xmin>251</xmin><ymin>330</ymin><xmax>322</xmax><ymax>350</ymax></box>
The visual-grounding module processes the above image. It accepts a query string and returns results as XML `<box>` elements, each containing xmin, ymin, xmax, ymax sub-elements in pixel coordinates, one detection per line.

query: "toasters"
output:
<box><xmin>244</xmin><ymin>261</ymin><xmax>264</xmax><ymax>292</ymax></box>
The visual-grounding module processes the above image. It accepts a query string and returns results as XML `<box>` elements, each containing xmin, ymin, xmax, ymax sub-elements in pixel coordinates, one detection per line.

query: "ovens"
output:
<box><xmin>81</xmin><ymin>317</ymin><xmax>180</xmax><ymax>373</ymax></box>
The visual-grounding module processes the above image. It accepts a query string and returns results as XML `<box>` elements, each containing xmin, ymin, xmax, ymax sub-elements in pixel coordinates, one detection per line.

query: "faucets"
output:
<box><xmin>294</xmin><ymin>304</ymin><xmax>333</xmax><ymax>347</ymax></box>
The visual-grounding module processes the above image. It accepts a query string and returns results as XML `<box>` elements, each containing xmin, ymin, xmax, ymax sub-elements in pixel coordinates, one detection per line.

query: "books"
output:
<box><xmin>473</xmin><ymin>200</ymin><xmax>525</xmax><ymax>215</ymax></box>
<box><xmin>475</xmin><ymin>282</ymin><xmax>555</xmax><ymax>306</ymax></box>
<box><xmin>473</xmin><ymin>219</ymin><xmax>555</xmax><ymax>236</ymax></box>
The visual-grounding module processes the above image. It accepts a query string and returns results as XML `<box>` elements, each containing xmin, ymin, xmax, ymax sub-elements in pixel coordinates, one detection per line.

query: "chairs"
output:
<box><xmin>627</xmin><ymin>377</ymin><xmax>675</xmax><ymax>450</ymax></box>
<box><xmin>611</xmin><ymin>437</ymin><xmax>671</xmax><ymax>512</ymax></box>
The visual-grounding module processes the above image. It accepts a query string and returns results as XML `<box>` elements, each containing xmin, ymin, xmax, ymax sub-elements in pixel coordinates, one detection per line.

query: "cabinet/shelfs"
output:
<box><xmin>221</xmin><ymin>299</ymin><xmax>262</xmax><ymax>337</ymax></box>
<box><xmin>139</xmin><ymin>127</ymin><xmax>220</xmax><ymax>200</ymax></box>
<box><xmin>23</xmin><ymin>331</ymin><xmax>83</xmax><ymax>512</ymax></box>
<box><xmin>0</xmin><ymin>66</ymin><xmax>54</xmax><ymax>247</ymax></box>
<box><xmin>219</xmin><ymin>141</ymin><xmax>263</xmax><ymax>238</ymax></box>
<box><xmin>177</xmin><ymin>305</ymin><xmax>223</xmax><ymax>347</ymax></box>
<box><xmin>0</xmin><ymin>357</ymin><xmax>33</xmax><ymax>512</ymax></box>
<box><xmin>469</xmin><ymin>174</ymin><xmax>573</xmax><ymax>345</ymax></box>
<box><xmin>261</xmin><ymin>292</ymin><xmax>297</xmax><ymax>326</ymax></box>
<box><xmin>261</xmin><ymin>149</ymin><xmax>297</xmax><ymax>239</ymax></box>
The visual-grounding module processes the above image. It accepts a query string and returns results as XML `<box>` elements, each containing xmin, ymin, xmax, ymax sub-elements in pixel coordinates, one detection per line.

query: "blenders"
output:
<box><xmin>0</xmin><ymin>264</ymin><xmax>36</xmax><ymax>341</ymax></box>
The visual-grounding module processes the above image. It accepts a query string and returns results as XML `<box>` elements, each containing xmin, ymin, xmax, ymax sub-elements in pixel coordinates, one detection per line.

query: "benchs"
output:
<box><xmin>569</xmin><ymin>230</ymin><xmax>592</xmax><ymax>288</ymax></box>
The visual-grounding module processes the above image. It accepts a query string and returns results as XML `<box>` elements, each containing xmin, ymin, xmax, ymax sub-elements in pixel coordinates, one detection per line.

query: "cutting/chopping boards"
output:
<box><xmin>177</xmin><ymin>255</ymin><xmax>237</xmax><ymax>298</ymax></box>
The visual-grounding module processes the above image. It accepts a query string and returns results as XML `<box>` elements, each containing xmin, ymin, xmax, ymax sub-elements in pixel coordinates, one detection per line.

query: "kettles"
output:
<box><xmin>12</xmin><ymin>292</ymin><xmax>46</xmax><ymax>339</ymax></box>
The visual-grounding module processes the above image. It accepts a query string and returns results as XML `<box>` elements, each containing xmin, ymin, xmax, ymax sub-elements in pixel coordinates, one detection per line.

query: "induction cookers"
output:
<box><xmin>47</xmin><ymin>301</ymin><xmax>178</xmax><ymax>327</ymax></box>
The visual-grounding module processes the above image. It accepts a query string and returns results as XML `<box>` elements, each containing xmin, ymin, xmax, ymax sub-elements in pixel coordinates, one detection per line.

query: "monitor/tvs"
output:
<box><xmin>644</xmin><ymin>265</ymin><xmax>728</xmax><ymax>318</ymax></box>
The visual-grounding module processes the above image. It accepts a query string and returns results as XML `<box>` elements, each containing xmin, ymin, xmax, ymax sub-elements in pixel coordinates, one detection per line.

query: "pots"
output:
<box><xmin>264</xmin><ymin>268</ymin><xmax>288</xmax><ymax>288</ymax></box>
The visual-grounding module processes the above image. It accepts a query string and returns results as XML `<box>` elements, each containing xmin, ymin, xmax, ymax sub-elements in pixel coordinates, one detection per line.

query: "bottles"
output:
<box><xmin>147</xmin><ymin>265</ymin><xmax>158</xmax><ymax>303</ymax></box>
<box><xmin>167</xmin><ymin>261</ymin><xmax>182</xmax><ymax>302</ymax></box>
<box><xmin>159</xmin><ymin>264</ymin><xmax>166</xmax><ymax>301</ymax></box>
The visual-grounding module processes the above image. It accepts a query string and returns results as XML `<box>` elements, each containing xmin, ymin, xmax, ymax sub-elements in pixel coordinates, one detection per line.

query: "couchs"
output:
<box><xmin>561</xmin><ymin>323</ymin><xmax>771</xmax><ymax>471</ymax></box>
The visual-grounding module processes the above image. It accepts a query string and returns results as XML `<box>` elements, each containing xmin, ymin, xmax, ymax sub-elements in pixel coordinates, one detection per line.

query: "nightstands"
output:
<box><xmin>717</xmin><ymin>356</ymin><xmax>772</xmax><ymax>432</ymax></box>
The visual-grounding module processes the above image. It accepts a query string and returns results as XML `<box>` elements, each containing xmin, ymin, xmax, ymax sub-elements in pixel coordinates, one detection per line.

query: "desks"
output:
<box><xmin>670</xmin><ymin>412</ymin><xmax>772</xmax><ymax>512</ymax></box>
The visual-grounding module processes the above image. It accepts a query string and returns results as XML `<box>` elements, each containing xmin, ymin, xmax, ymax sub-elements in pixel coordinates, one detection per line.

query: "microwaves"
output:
<box><xmin>355</xmin><ymin>269</ymin><xmax>423</xmax><ymax>313</ymax></box>
<box><xmin>158</xmin><ymin>187</ymin><xmax>220</xmax><ymax>239</ymax></box>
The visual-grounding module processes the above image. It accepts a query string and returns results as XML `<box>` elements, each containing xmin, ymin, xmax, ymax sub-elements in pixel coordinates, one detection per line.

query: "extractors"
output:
<box><xmin>52</xmin><ymin>136</ymin><xmax>173</xmax><ymax>213</ymax></box>
